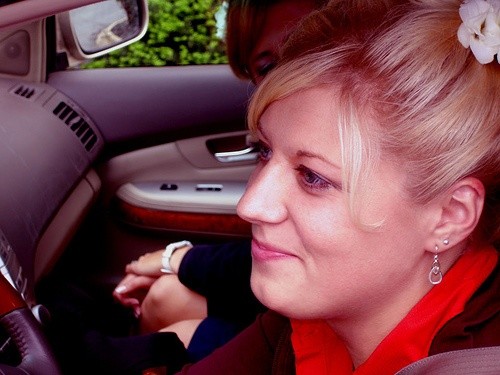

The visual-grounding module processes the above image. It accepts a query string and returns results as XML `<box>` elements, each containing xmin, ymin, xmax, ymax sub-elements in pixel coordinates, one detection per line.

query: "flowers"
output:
<box><xmin>455</xmin><ymin>0</ymin><xmax>500</xmax><ymax>65</ymax></box>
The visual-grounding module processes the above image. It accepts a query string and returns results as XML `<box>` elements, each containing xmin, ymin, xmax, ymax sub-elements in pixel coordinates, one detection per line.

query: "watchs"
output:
<box><xmin>158</xmin><ymin>241</ymin><xmax>194</xmax><ymax>273</ymax></box>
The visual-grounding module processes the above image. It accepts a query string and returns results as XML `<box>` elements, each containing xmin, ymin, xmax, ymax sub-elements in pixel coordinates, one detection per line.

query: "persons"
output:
<box><xmin>226</xmin><ymin>1</ymin><xmax>500</xmax><ymax>375</ymax></box>
<box><xmin>59</xmin><ymin>237</ymin><xmax>265</xmax><ymax>375</ymax></box>
